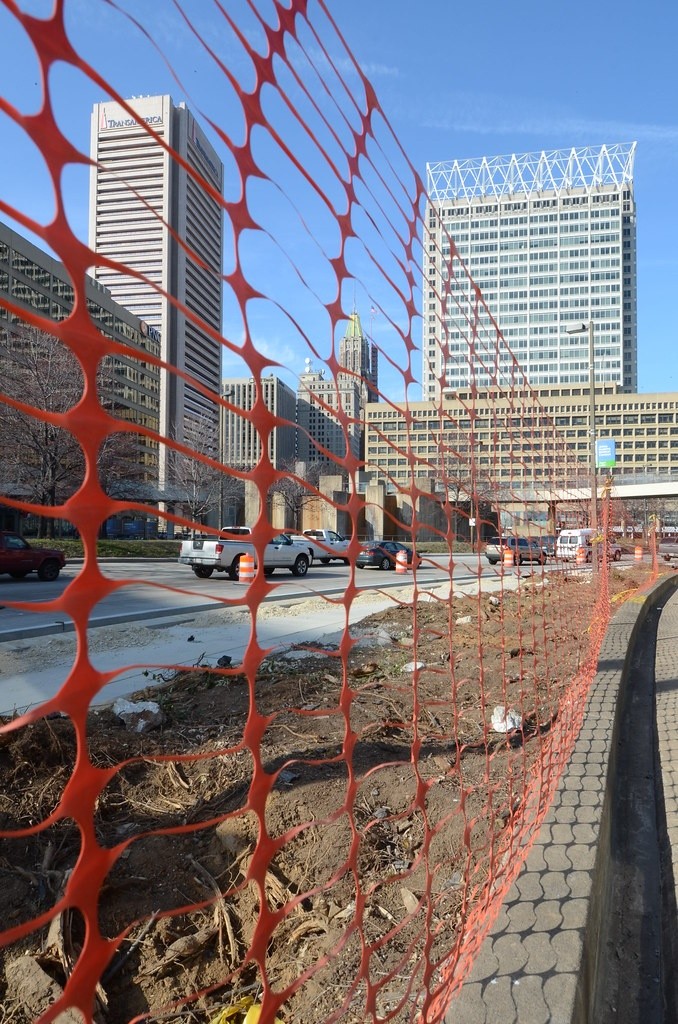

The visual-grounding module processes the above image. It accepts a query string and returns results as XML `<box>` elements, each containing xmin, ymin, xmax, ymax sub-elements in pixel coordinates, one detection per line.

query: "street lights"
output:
<box><xmin>565</xmin><ymin>320</ymin><xmax>597</xmax><ymax>574</ymax></box>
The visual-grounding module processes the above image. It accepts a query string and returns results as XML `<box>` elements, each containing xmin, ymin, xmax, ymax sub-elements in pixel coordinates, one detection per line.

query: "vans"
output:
<box><xmin>554</xmin><ymin>528</ymin><xmax>621</xmax><ymax>565</ymax></box>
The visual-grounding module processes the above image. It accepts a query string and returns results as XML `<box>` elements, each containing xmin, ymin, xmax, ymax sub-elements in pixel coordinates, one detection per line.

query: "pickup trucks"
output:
<box><xmin>178</xmin><ymin>526</ymin><xmax>312</xmax><ymax>581</ymax></box>
<box><xmin>290</xmin><ymin>529</ymin><xmax>351</xmax><ymax>565</ymax></box>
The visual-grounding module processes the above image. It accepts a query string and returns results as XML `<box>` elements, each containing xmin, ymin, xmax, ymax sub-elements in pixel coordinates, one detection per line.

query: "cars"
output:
<box><xmin>356</xmin><ymin>541</ymin><xmax>421</xmax><ymax>570</ymax></box>
<box><xmin>0</xmin><ymin>531</ymin><xmax>66</xmax><ymax>582</ymax></box>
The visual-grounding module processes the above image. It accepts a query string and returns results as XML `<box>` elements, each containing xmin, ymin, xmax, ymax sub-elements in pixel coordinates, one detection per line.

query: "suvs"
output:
<box><xmin>484</xmin><ymin>536</ymin><xmax>547</xmax><ymax>566</ymax></box>
<box><xmin>658</xmin><ymin>536</ymin><xmax>678</xmax><ymax>561</ymax></box>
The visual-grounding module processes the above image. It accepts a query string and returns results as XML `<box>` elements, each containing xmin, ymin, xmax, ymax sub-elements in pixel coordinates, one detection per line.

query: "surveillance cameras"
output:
<box><xmin>566</xmin><ymin>323</ymin><xmax>586</xmax><ymax>334</ymax></box>
